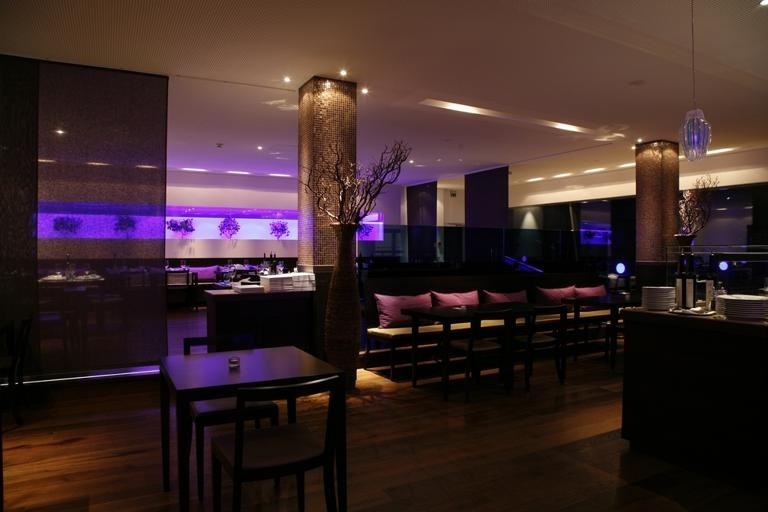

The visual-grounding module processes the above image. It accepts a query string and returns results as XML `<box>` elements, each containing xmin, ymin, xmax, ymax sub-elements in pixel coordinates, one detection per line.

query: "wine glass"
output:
<box><xmin>228</xmin><ymin>266</ymin><xmax>236</xmax><ymax>285</ymax></box>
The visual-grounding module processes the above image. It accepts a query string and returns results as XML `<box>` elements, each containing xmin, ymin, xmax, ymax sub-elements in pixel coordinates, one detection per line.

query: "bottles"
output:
<box><xmin>263</xmin><ymin>253</ymin><xmax>285</xmax><ymax>274</ymax></box>
<box><xmin>676</xmin><ymin>252</ymin><xmax>695</xmax><ymax>309</ymax></box>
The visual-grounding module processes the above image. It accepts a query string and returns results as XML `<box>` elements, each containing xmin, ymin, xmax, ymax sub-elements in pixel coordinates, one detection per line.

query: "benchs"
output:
<box><xmin>365</xmin><ymin>274</ymin><xmax>611</xmax><ymax>380</ymax></box>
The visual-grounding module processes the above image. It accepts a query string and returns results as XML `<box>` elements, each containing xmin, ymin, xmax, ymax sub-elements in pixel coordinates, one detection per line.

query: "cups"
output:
<box><xmin>229</xmin><ymin>356</ymin><xmax>240</xmax><ymax>370</ymax></box>
<box><xmin>223</xmin><ymin>274</ymin><xmax>230</xmax><ymax>285</ymax></box>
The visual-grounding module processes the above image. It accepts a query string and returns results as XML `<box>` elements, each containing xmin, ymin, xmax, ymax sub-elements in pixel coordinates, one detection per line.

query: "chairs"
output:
<box><xmin>448</xmin><ymin>295</ymin><xmax>618</xmax><ymax>404</ymax></box>
<box><xmin>210</xmin><ymin>375</ymin><xmax>348</xmax><ymax>512</ymax></box>
<box><xmin>0</xmin><ymin>317</ymin><xmax>34</xmax><ymax>426</ymax></box>
<box><xmin>176</xmin><ymin>334</ymin><xmax>280</xmax><ymax>511</ymax></box>
<box><xmin>38</xmin><ymin>266</ymin><xmax>259</xmax><ymax>339</ymax></box>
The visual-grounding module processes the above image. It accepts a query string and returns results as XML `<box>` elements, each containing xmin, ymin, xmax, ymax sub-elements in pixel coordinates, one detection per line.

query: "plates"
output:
<box><xmin>716</xmin><ymin>295</ymin><xmax>767</xmax><ymax>317</ymax></box>
<box><xmin>642</xmin><ymin>285</ymin><xmax>676</xmax><ymax>311</ymax></box>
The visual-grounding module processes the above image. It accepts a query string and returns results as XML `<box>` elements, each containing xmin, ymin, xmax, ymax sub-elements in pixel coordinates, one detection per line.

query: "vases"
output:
<box><xmin>672</xmin><ymin>232</ymin><xmax>697</xmax><ymax>310</ymax></box>
<box><xmin>321</xmin><ymin>221</ymin><xmax>363</xmax><ymax>394</ymax></box>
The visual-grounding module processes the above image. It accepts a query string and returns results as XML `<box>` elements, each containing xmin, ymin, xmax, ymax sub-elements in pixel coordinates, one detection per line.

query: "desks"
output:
<box><xmin>160</xmin><ymin>345</ymin><xmax>346</xmax><ymax>511</ymax></box>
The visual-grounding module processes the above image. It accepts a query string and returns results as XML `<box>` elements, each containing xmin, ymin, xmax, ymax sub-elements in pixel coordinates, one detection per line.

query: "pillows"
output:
<box><xmin>371</xmin><ymin>281</ymin><xmax>609</xmax><ymax>329</ymax></box>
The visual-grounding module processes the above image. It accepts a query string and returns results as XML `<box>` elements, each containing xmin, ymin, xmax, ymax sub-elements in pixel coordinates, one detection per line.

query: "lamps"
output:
<box><xmin>678</xmin><ymin>0</ymin><xmax>713</xmax><ymax>161</ymax></box>
<box><xmin>51</xmin><ymin>211</ymin><xmax>288</xmax><ymax>240</ymax></box>
